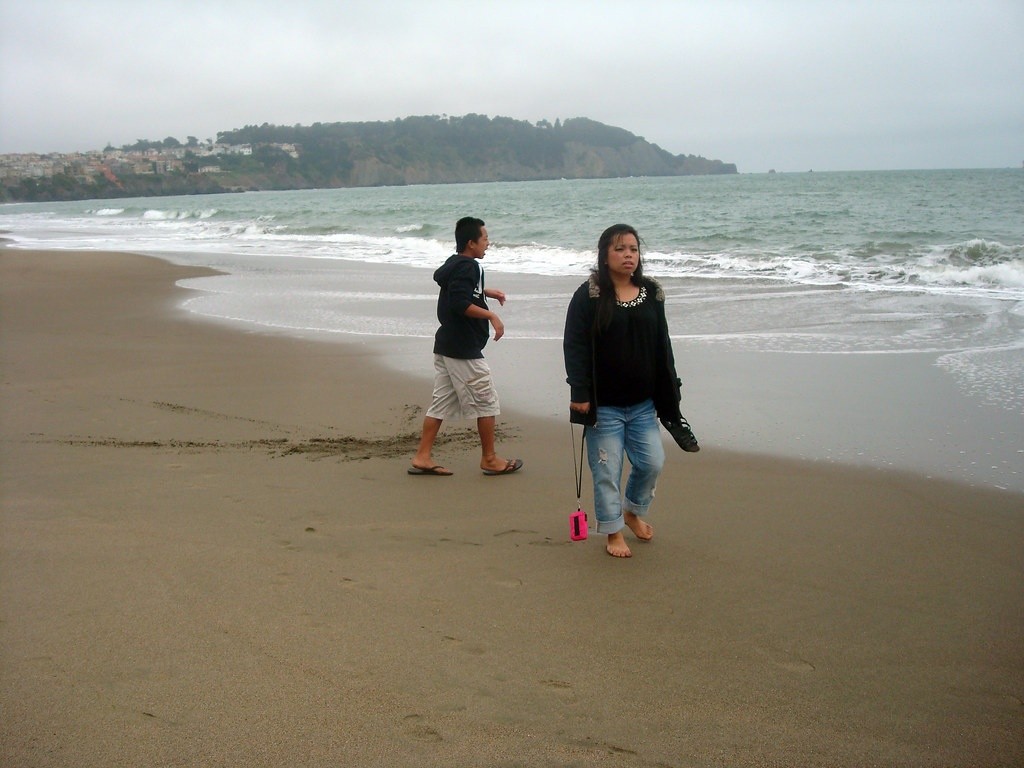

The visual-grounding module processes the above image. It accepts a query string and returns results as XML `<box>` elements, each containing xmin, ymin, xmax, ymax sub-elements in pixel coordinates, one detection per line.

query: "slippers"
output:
<box><xmin>408</xmin><ymin>465</ymin><xmax>453</xmax><ymax>476</ymax></box>
<box><xmin>483</xmin><ymin>459</ymin><xmax>523</xmax><ymax>475</ymax></box>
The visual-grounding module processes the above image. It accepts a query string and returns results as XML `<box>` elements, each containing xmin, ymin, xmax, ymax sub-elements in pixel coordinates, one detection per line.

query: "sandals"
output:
<box><xmin>660</xmin><ymin>416</ymin><xmax>695</xmax><ymax>452</ymax></box>
<box><xmin>679</xmin><ymin>413</ymin><xmax>700</xmax><ymax>451</ymax></box>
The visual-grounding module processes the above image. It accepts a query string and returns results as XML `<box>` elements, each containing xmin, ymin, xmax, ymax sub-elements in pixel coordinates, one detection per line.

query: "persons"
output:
<box><xmin>561</xmin><ymin>223</ymin><xmax>684</xmax><ymax>559</ymax></box>
<box><xmin>407</xmin><ymin>217</ymin><xmax>523</xmax><ymax>476</ymax></box>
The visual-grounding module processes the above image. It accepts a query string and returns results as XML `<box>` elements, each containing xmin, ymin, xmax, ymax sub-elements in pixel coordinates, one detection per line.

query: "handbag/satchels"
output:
<box><xmin>570</xmin><ymin>512</ymin><xmax>588</xmax><ymax>541</ymax></box>
